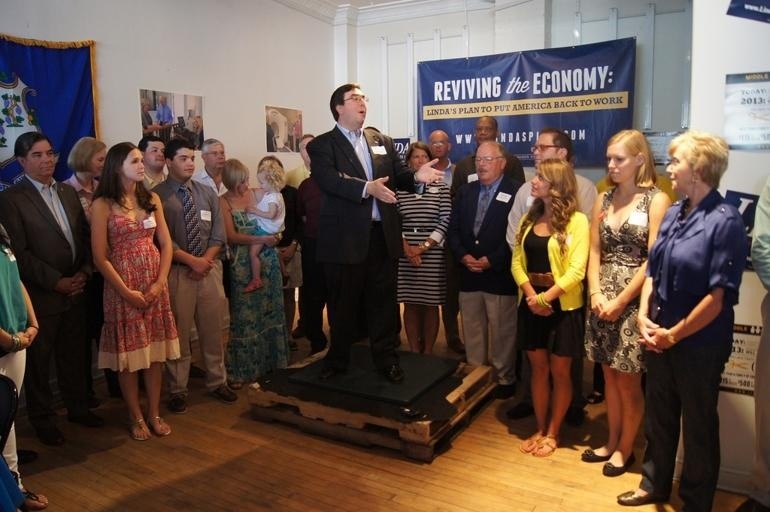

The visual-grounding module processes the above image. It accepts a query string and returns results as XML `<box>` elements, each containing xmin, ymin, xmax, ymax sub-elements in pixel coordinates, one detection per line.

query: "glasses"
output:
<box><xmin>474</xmin><ymin>156</ymin><xmax>505</xmax><ymax>162</ymax></box>
<box><xmin>530</xmin><ymin>144</ymin><xmax>560</xmax><ymax>152</ymax></box>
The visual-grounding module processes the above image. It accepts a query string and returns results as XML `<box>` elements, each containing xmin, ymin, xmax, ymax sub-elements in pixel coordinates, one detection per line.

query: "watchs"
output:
<box><xmin>424</xmin><ymin>241</ymin><xmax>432</xmax><ymax>248</ymax></box>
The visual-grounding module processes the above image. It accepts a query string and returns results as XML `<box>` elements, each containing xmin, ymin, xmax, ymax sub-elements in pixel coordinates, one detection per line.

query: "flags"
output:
<box><xmin>0</xmin><ymin>33</ymin><xmax>101</xmax><ymax>190</ymax></box>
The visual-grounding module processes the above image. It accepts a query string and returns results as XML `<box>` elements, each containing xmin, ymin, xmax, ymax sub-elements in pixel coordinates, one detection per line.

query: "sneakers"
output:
<box><xmin>242</xmin><ymin>278</ymin><xmax>263</xmax><ymax>292</ymax></box>
<box><xmin>293</xmin><ymin>325</ymin><xmax>305</xmax><ymax>339</ymax></box>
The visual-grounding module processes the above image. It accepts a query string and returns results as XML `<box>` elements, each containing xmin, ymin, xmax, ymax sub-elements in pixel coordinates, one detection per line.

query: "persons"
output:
<box><xmin>616</xmin><ymin>131</ymin><xmax>746</xmax><ymax>511</ymax></box>
<box><xmin>306</xmin><ymin>84</ymin><xmax>447</xmax><ymax>386</ymax></box>
<box><xmin>141</xmin><ymin>96</ymin><xmax>173</xmax><ymax>143</ymax></box>
<box><xmin>366</xmin><ymin>116</ymin><xmax>680</xmax><ymax>430</ymax></box>
<box><xmin>510</xmin><ymin>157</ymin><xmax>590</xmax><ymax>457</ymax></box>
<box><xmin>1</xmin><ymin>134</ymin><xmax>328</xmax><ymax>511</ymax></box>
<box><xmin>735</xmin><ymin>174</ymin><xmax>769</xmax><ymax>511</ymax></box>
<box><xmin>580</xmin><ymin>130</ymin><xmax>672</xmax><ymax>477</ymax></box>
<box><xmin>266</xmin><ymin>108</ymin><xmax>302</xmax><ymax>152</ymax></box>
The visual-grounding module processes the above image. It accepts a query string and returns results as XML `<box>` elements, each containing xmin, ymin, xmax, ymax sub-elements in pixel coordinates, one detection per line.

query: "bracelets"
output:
<box><xmin>538</xmin><ymin>293</ymin><xmax>545</xmax><ymax>306</ymax></box>
<box><xmin>13</xmin><ymin>335</ymin><xmax>22</xmax><ymax>352</ymax></box>
<box><xmin>537</xmin><ymin>296</ymin><xmax>541</xmax><ymax>305</ymax></box>
<box><xmin>419</xmin><ymin>243</ymin><xmax>428</xmax><ymax>250</ymax></box>
<box><xmin>590</xmin><ymin>291</ymin><xmax>602</xmax><ymax>296</ymax></box>
<box><xmin>31</xmin><ymin>326</ymin><xmax>39</xmax><ymax>331</ymax></box>
<box><xmin>541</xmin><ymin>292</ymin><xmax>549</xmax><ymax>305</ymax></box>
<box><xmin>668</xmin><ymin>330</ymin><xmax>676</xmax><ymax>344</ymax></box>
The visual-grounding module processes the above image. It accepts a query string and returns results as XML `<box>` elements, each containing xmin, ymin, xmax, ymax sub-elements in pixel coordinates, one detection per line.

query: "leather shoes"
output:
<box><xmin>564</xmin><ymin>390</ymin><xmax>603</xmax><ymax>426</ymax></box>
<box><xmin>168</xmin><ymin>398</ymin><xmax>185</xmax><ymax>413</ymax></box>
<box><xmin>494</xmin><ymin>382</ymin><xmax>534</xmax><ymax>420</ymax></box>
<box><xmin>211</xmin><ymin>383</ymin><xmax>237</xmax><ymax>402</ymax></box>
<box><xmin>36</xmin><ymin>427</ymin><xmax>64</xmax><ymax>446</ymax></box>
<box><xmin>618</xmin><ymin>490</ymin><xmax>670</xmax><ymax>506</ymax></box>
<box><xmin>383</xmin><ymin>362</ymin><xmax>405</xmax><ymax>385</ymax></box>
<box><xmin>603</xmin><ymin>450</ymin><xmax>635</xmax><ymax>476</ymax></box>
<box><xmin>17</xmin><ymin>449</ymin><xmax>37</xmax><ymax>465</ymax></box>
<box><xmin>320</xmin><ymin>363</ymin><xmax>333</xmax><ymax>379</ymax></box>
<box><xmin>66</xmin><ymin>410</ymin><xmax>103</xmax><ymax>428</ymax></box>
<box><xmin>582</xmin><ymin>448</ymin><xmax>612</xmax><ymax>462</ymax></box>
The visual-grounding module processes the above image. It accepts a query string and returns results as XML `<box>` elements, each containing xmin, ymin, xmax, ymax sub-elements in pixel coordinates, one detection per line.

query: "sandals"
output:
<box><xmin>20</xmin><ymin>488</ymin><xmax>48</xmax><ymax>510</ymax></box>
<box><xmin>127</xmin><ymin>417</ymin><xmax>151</xmax><ymax>441</ymax></box>
<box><xmin>147</xmin><ymin>414</ymin><xmax>171</xmax><ymax>436</ymax></box>
<box><xmin>519</xmin><ymin>435</ymin><xmax>560</xmax><ymax>457</ymax></box>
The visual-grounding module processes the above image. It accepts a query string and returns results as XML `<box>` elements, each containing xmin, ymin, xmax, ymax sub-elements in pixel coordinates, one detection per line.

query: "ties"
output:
<box><xmin>180</xmin><ymin>184</ymin><xmax>203</xmax><ymax>257</ymax></box>
<box><xmin>474</xmin><ymin>184</ymin><xmax>492</xmax><ymax>236</ymax></box>
